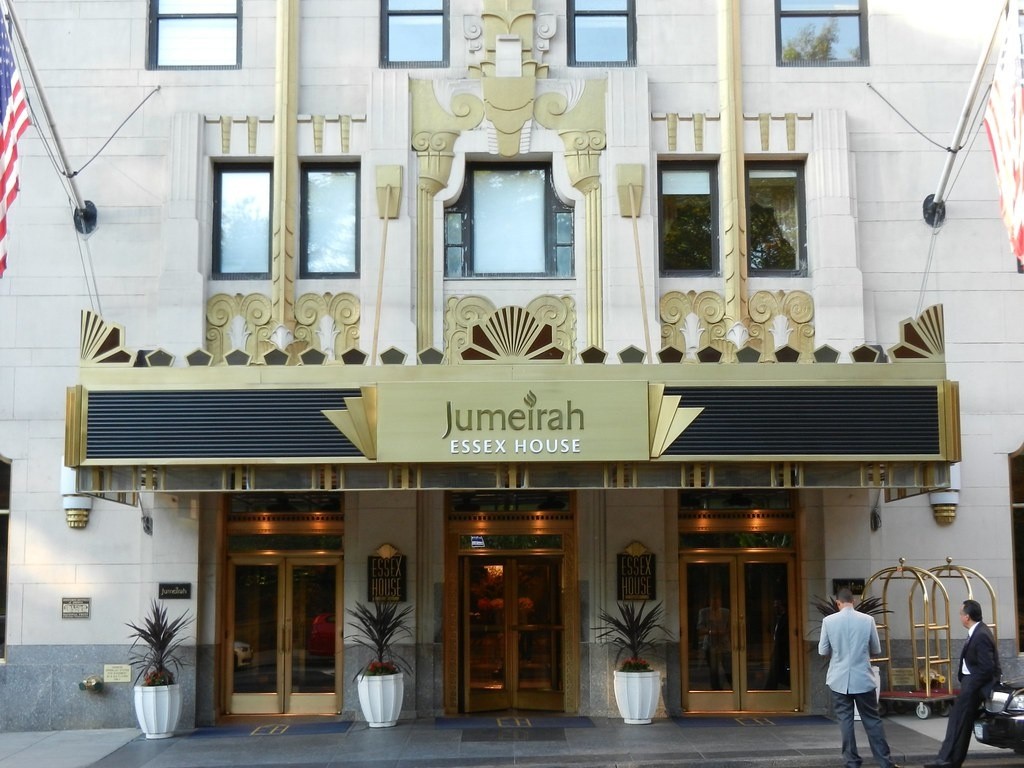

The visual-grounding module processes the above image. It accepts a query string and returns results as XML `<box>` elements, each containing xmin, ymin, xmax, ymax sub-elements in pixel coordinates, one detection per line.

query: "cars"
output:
<box><xmin>972</xmin><ymin>676</ymin><xmax>1024</xmax><ymax>756</ymax></box>
<box><xmin>234</xmin><ymin>641</ymin><xmax>255</xmax><ymax>671</ymax></box>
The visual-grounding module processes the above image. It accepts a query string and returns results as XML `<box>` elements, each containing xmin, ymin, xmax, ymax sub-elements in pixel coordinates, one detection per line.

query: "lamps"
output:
<box><xmin>376</xmin><ymin>543</ymin><xmax>399</xmax><ymax>561</ymax></box>
<box><xmin>624</xmin><ymin>542</ymin><xmax>649</xmax><ymax>562</ymax></box>
<box><xmin>79</xmin><ymin>675</ymin><xmax>104</xmax><ymax>695</ymax></box>
<box><xmin>61</xmin><ymin>455</ymin><xmax>92</xmax><ymax>528</ymax></box>
<box><xmin>929</xmin><ymin>462</ymin><xmax>960</xmax><ymax>527</ymax></box>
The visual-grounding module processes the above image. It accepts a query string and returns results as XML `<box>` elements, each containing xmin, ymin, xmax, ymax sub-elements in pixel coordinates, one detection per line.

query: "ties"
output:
<box><xmin>965</xmin><ymin>634</ymin><xmax>970</xmax><ymax>644</ymax></box>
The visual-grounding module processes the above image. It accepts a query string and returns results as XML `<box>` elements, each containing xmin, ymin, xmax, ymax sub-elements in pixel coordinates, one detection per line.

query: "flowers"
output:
<box><xmin>621</xmin><ymin>659</ymin><xmax>651</xmax><ymax>672</ymax></box>
<box><xmin>364</xmin><ymin>661</ymin><xmax>397</xmax><ymax>676</ymax></box>
<box><xmin>143</xmin><ymin>670</ymin><xmax>173</xmax><ymax>686</ymax></box>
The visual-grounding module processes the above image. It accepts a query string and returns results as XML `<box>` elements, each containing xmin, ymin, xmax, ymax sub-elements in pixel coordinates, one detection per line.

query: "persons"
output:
<box><xmin>818</xmin><ymin>586</ymin><xmax>901</xmax><ymax>768</ymax></box>
<box><xmin>697</xmin><ymin>591</ymin><xmax>732</xmax><ymax>691</ymax></box>
<box><xmin>923</xmin><ymin>599</ymin><xmax>1002</xmax><ymax>768</ymax></box>
<box><xmin>768</xmin><ymin>598</ymin><xmax>790</xmax><ymax>690</ymax></box>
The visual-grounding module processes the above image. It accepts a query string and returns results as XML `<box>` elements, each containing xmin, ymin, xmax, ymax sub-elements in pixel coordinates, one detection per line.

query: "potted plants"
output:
<box><xmin>344</xmin><ymin>592</ymin><xmax>417</xmax><ymax>727</ymax></box>
<box><xmin>589</xmin><ymin>598</ymin><xmax>677</xmax><ymax>724</ymax></box>
<box><xmin>124</xmin><ymin>597</ymin><xmax>197</xmax><ymax>739</ymax></box>
<box><xmin>804</xmin><ymin>594</ymin><xmax>896</xmax><ymax>721</ymax></box>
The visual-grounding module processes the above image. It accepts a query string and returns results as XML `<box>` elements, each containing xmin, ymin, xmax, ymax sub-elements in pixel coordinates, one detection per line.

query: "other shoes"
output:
<box><xmin>924</xmin><ymin>758</ymin><xmax>961</xmax><ymax>768</ymax></box>
<box><xmin>890</xmin><ymin>763</ymin><xmax>900</xmax><ymax>768</ymax></box>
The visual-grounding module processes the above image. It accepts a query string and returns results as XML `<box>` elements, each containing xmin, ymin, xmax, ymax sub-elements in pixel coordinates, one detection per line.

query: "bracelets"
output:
<box><xmin>709</xmin><ymin>629</ymin><xmax>712</xmax><ymax>634</ymax></box>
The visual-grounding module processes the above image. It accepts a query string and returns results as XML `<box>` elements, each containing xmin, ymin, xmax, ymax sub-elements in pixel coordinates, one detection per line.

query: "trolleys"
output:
<box><xmin>910</xmin><ymin>556</ymin><xmax>998</xmax><ymax>696</ymax></box>
<box><xmin>859</xmin><ymin>557</ymin><xmax>956</xmax><ymax>720</ymax></box>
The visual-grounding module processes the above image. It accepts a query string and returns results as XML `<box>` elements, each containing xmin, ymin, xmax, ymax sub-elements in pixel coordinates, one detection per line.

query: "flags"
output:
<box><xmin>0</xmin><ymin>5</ymin><xmax>34</xmax><ymax>279</ymax></box>
<box><xmin>982</xmin><ymin>0</ymin><xmax>1024</xmax><ymax>277</ymax></box>
